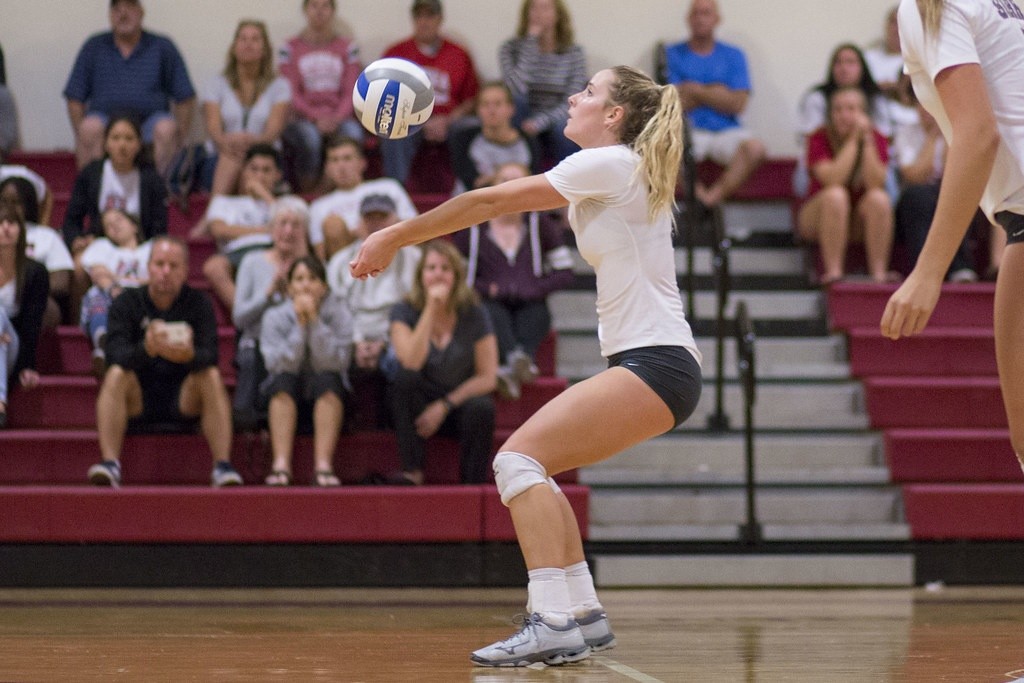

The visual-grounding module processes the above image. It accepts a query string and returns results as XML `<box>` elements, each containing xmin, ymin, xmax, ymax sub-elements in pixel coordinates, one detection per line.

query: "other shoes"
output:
<box><xmin>89</xmin><ymin>460</ymin><xmax>122</xmax><ymax>485</ymax></box>
<box><xmin>266</xmin><ymin>470</ymin><xmax>289</xmax><ymax>487</ymax></box>
<box><xmin>211</xmin><ymin>461</ymin><xmax>242</xmax><ymax>485</ymax></box>
<box><xmin>317</xmin><ymin>472</ymin><xmax>338</xmax><ymax>488</ymax></box>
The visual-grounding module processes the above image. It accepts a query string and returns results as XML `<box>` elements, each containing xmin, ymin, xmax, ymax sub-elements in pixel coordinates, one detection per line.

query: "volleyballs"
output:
<box><xmin>352</xmin><ymin>58</ymin><xmax>437</xmax><ymax>141</ymax></box>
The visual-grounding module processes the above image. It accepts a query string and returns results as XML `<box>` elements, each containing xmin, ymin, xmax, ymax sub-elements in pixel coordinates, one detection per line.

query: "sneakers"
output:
<box><xmin>470</xmin><ymin>612</ymin><xmax>591</xmax><ymax>667</ymax></box>
<box><xmin>574</xmin><ymin>610</ymin><xmax>616</xmax><ymax>652</ymax></box>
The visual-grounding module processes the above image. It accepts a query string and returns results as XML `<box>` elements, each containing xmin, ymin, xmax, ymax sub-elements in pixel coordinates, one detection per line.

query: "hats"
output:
<box><xmin>363</xmin><ymin>193</ymin><xmax>396</xmax><ymax>216</ymax></box>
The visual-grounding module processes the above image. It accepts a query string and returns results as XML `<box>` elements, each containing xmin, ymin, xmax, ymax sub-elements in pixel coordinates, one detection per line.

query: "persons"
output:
<box><xmin>448</xmin><ymin>81</ymin><xmax>537</xmax><ymax>189</ymax></box>
<box><xmin>880</xmin><ymin>1</ymin><xmax>1024</xmax><ymax>470</ymax></box>
<box><xmin>500</xmin><ymin>0</ymin><xmax>587</xmax><ymax>174</ymax></box>
<box><xmin>349</xmin><ymin>64</ymin><xmax>702</xmax><ymax>671</ymax></box>
<box><xmin>794</xmin><ymin>6</ymin><xmax>1008</xmax><ymax>284</ymax></box>
<box><xmin>63</xmin><ymin>0</ymin><xmax>197</xmax><ymax>181</ymax></box>
<box><xmin>279</xmin><ymin>0</ymin><xmax>365</xmax><ymax>193</ymax></box>
<box><xmin>389</xmin><ymin>241</ymin><xmax>499</xmax><ymax>485</ymax></box>
<box><xmin>794</xmin><ymin>88</ymin><xmax>894</xmax><ymax>284</ymax></box>
<box><xmin>0</xmin><ymin>122</ymin><xmax>575</xmax><ymax>435</ymax></box>
<box><xmin>261</xmin><ymin>255</ymin><xmax>355</xmax><ymax>488</ymax></box>
<box><xmin>184</xmin><ymin>20</ymin><xmax>294</xmax><ymax>237</ymax></box>
<box><xmin>664</xmin><ymin>0</ymin><xmax>764</xmax><ymax>204</ymax></box>
<box><xmin>379</xmin><ymin>0</ymin><xmax>479</xmax><ymax>186</ymax></box>
<box><xmin>450</xmin><ymin>160</ymin><xmax>576</xmax><ymax>401</ymax></box>
<box><xmin>0</xmin><ymin>46</ymin><xmax>21</xmax><ymax>163</ymax></box>
<box><xmin>83</xmin><ymin>234</ymin><xmax>246</xmax><ymax>487</ymax></box>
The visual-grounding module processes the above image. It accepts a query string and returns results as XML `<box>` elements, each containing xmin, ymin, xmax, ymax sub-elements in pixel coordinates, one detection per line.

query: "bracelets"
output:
<box><xmin>440</xmin><ymin>395</ymin><xmax>457</xmax><ymax>413</ymax></box>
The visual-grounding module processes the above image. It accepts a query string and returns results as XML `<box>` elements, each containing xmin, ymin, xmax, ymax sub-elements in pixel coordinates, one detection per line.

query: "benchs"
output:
<box><xmin>0</xmin><ymin>146</ymin><xmax>1024</xmax><ymax>591</ymax></box>
